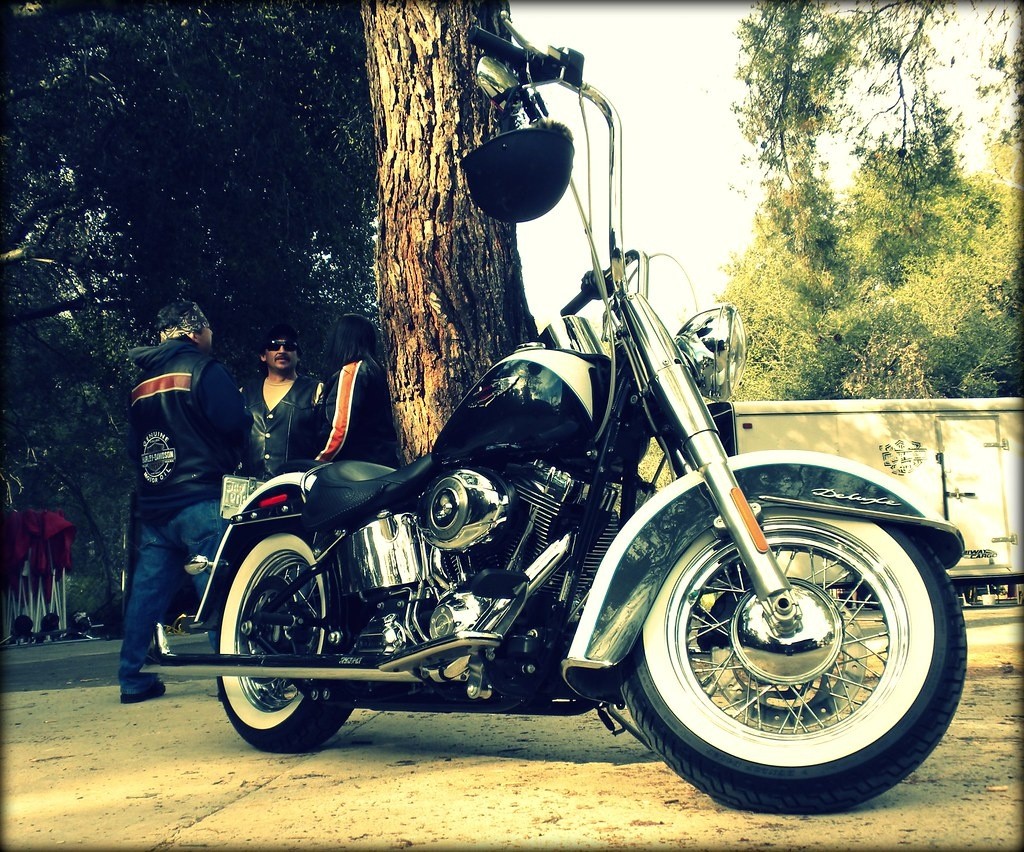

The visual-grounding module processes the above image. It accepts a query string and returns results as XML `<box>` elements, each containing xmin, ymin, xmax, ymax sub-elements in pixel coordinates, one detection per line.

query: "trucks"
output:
<box><xmin>704</xmin><ymin>397</ymin><xmax>1023</xmax><ymax>581</ymax></box>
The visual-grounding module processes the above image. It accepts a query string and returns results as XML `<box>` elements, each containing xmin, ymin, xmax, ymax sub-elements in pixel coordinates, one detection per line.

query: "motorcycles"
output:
<box><xmin>138</xmin><ymin>10</ymin><xmax>966</xmax><ymax>816</ymax></box>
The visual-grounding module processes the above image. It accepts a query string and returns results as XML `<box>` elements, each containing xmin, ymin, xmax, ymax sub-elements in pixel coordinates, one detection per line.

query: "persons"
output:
<box><xmin>118</xmin><ymin>300</ymin><xmax>255</xmax><ymax>704</ymax></box>
<box><xmin>307</xmin><ymin>312</ymin><xmax>403</xmax><ymax>471</ymax></box>
<box><xmin>230</xmin><ymin>318</ymin><xmax>330</xmax><ymax>485</ymax></box>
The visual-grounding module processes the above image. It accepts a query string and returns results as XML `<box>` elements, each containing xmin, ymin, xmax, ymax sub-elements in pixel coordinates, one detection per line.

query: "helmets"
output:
<box><xmin>459</xmin><ymin>128</ymin><xmax>575</xmax><ymax>224</ymax></box>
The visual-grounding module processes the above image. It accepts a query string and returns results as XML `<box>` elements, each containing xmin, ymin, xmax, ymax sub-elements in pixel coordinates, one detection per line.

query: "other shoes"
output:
<box><xmin>120</xmin><ymin>681</ymin><xmax>166</xmax><ymax>704</ymax></box>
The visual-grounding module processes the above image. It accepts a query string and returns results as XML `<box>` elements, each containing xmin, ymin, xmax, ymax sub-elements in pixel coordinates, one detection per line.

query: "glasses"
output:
<box><xmin>267</xmin><ymin>339</ymin><xmax>297</xmax><ymax>351</ymax></box>
